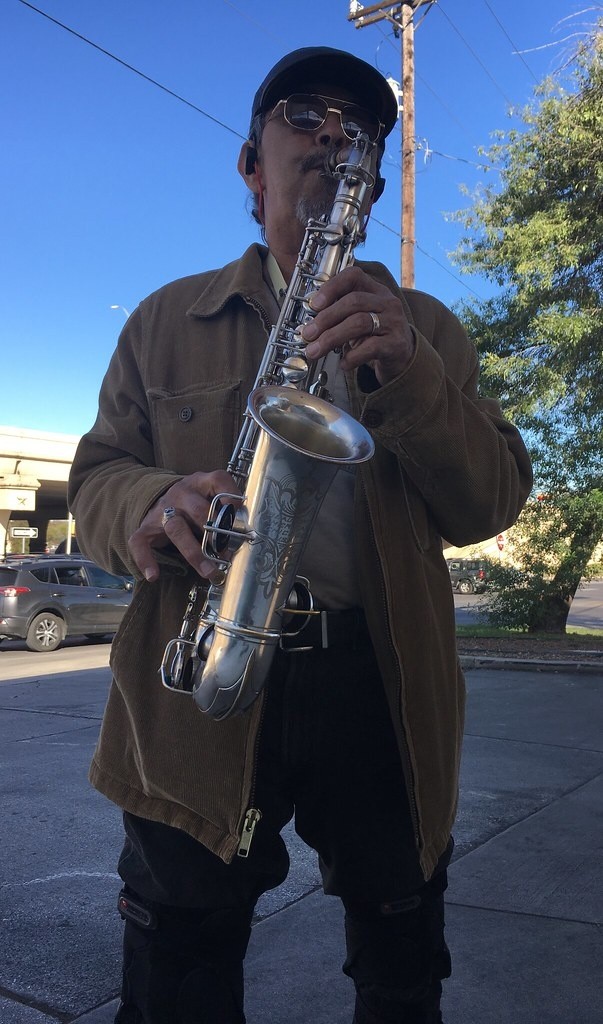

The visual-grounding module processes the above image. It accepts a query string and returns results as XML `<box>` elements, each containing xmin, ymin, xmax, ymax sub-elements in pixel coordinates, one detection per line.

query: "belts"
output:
<box><xmin>277</xmin><ymin>607</ymin><xmax>368</xmax><ymax>651</ymax></box>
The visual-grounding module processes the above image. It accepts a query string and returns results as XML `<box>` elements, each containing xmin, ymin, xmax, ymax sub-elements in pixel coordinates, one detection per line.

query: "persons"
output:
<box><xmin>67</xmin><ymin>46</ymin><xmax>533</xmax><ymax>1024</ymax></box>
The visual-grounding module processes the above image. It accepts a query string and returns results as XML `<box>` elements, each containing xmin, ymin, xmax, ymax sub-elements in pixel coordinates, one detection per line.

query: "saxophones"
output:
<box><xmin>150</xmin><ymin>129</ymin><xmax>377</xmax><ymax>720</ymax></box>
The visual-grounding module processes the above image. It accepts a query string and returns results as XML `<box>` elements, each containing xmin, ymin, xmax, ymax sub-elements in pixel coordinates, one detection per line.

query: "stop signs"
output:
<box><xmin>497</xmin><ymin>535</ymin><xmax>505</xmax><ymax>551</ymax></box>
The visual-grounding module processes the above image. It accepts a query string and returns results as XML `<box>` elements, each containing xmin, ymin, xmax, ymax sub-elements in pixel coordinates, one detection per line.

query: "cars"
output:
<box><xmin>54</xmin><ymin>536</ymin><xmax>81</xmax><ymax>554</ymax></box>
<box><xmin>444</xmin><ymin>557</ymin><xmax>491</xmax><ymax>595</ymax></box>
<box><xmin>0</xmin><ymin>551</ymin><xmax>137</xmax><ymax>652</ymax></box>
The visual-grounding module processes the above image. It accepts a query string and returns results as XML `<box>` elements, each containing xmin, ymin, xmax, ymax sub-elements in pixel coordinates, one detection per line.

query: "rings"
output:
<box><xmin>162</xmin><ymin>506</ymin><xmax>179</xmax><ymax>526</ymax></box>
<box><xmin>370</xmin><ymin>312</ymin><xmax>380</xmax><ymax>335</ymax></box>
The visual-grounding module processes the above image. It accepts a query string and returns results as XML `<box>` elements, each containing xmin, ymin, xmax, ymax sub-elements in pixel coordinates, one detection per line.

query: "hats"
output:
<box><xmin>250</xmin><ymin>46</ymin><xmax>399</xmax><ymax>136</ymax></box>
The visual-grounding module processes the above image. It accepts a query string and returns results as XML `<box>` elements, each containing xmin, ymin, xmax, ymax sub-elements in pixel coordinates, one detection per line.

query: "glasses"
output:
<box><xmin>263</xmin><ymin>92</ymin><xmax>386</xmax><ymax>145</ymax></box>
